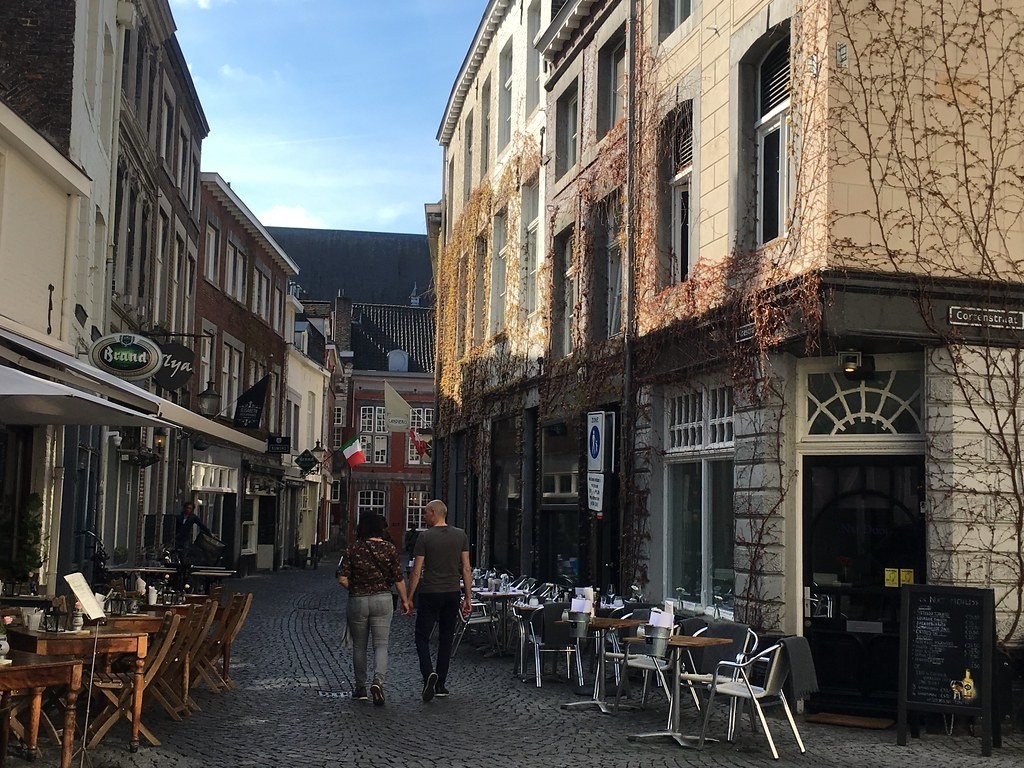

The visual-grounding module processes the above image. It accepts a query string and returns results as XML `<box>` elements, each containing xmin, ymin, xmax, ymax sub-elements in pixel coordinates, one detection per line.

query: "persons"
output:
<box><xmin>175</xmin><ymin>501</ymin><xmax>220</xmax><ymax>553</ymax></box>
<box><xmin>405</xmin><ymin>524</ymin><xmax>419</xmax><ymax>560</ymax></box>
<box><xmin>408</xmin><ymin>499</ymin><xmax>472</xmax><ymax>702</ymax></box>
<box><xmin>336</xmin><ymin>509</ymin><xmax>409</xmax><ymax>704</ymax></box>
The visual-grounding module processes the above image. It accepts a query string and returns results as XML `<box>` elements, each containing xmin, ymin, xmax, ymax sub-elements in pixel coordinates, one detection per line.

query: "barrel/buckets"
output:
<box><xmin>569</xmin><ymin>611</ymin><xmax>591</xmax><ymax>638</ymax></box>
<box><xmin>646</xmin><ymin>625</ymin><xmax>671</xmax><ymax>657</ymax></box>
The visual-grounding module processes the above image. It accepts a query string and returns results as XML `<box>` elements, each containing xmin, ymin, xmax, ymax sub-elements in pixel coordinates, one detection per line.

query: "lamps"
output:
<box><xmin>141</xmin><ymin>323</ymin><xmax>219</xmax><ymax>418</ymax></box>
<box><xmin>844</xmin><ymin>353</ymin><xmax>856</xmax><ymax>372</ymax></box>
<box><xmin>290</xmin><ymin>441</ymin><xmax>324</xmax><ymax>472</ymax></box>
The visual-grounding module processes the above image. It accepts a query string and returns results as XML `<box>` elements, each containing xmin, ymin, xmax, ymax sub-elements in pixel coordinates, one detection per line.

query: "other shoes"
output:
<box><xmin>435</xmin><ymin>688</ymin><xmax>450</xmax><ymax>697</ymax></box>
<box><xmin>353</xmin><ymin>687</ymin><xmax>368</xmax><ymax>700</ymax></box>
<box><xmin>370</xmin><ymin>683</ymin><xmax>385</xmax><ymax>707</ymax></box>
<box><xmin>423</xmin><ymin>672</ymin><xmax>439</xmax><ymax>702</ymax></box>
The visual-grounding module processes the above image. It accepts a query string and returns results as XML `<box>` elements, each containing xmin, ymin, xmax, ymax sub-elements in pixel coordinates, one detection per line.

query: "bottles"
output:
<box><xmin>488</xmin><ymin>576</ymin><xmax>493</xmax><ymax>591</ymax></box>
<box><xmin>963</xmin><ymin>671</ymin><xmax>973</xmax><ymax>698</ymax></box>
<box><xmin>530</xmin><ymin>595</ymin><xmax>538</xmax><ymax>606</ymax></box>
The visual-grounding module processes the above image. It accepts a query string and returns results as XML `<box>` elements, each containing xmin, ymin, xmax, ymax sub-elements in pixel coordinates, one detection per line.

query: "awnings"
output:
<box><xmin>0</xmin><ymin>329</ymin><xmax>268</xmax><ymax>455</ymax></box>
<box><xmin>0</xmin><ymin>364</ymin><xmax>183</xmax><ymax>428</ymax></box>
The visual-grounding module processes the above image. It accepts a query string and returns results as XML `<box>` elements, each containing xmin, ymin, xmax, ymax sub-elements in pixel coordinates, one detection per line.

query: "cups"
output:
<box><xmin>27</xmin><ymin>614</ymin><xmax>41</xmax><ymax>631</ymax></box>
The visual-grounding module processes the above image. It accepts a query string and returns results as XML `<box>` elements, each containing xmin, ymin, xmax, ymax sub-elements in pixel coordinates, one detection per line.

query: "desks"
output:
<box><xmin>510</xmin><ymin>605</ymin><xmax>545</xmax><ymax>682</ymax></box>
<box><xmin>479</xmin><ymin>591</ymin><xmax>527</xmax><ymax>657</ymax></box>
<box><xmin>7</xmin><ymin>626</ymin><xmax>148</xmax><ymax>761</ymax></box>
<box><xmin>90</xmin><ymin>612</ymin><xmax>190</xmax><ymax>715</ymax></box>
<box><xmin>555</xmin><ymin>618</ymin><xmax>650</xmax><ymax>713</ymax></box>
<box><xmin>622</xmin><ymin>635</ymin><xmax>733</xmax><ymax>749</ymax></box>
<box><xmin>0</xmin><ymin>650</ymin><xmax>84</xmax><ymax>768</ymax></box>
<box><xmin>143</xmin><ymin>602</ymin><xmax>210</xmax><ymax>670</ymax></box>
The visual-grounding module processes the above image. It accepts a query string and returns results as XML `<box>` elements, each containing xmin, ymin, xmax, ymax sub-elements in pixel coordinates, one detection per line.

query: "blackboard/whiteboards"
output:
<box><xmin>898</xmin><ymin>583</ymin><xmax>1000</xmax><ymax>715</ymax></box>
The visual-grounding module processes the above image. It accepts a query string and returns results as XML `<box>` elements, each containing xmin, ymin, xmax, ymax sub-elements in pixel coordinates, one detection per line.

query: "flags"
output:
<box><xmin>341</xmin><ymin>434</ymin><xmax>365</xmax><ymax>467</ymax></box>
<box><xmin>233</xmin><ymin>373</ymin><xmax>270</xmax><ymax>429</ymax></box>
<box><xmin>411</xmin><ymin>427</ymin><xmax>427</xmax><ymax>458</ymax></box>
<box><xmin>384</xmin><ymin>381</ymin><xmax>410</xmax><ymax>433</ymax></box>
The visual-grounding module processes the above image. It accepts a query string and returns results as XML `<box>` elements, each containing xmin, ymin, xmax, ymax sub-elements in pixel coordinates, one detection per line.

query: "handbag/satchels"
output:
<box><xmin>393</xmin><ymin>594</ymin><xmax>398</xmax><ymax>611</ymax></box>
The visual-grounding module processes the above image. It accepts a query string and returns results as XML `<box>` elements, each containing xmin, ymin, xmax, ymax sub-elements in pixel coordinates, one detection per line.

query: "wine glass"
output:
<box><xmin>523</xmin><ymin>589</ymin><xmax>531</xmax><ymax>606</ymax></box>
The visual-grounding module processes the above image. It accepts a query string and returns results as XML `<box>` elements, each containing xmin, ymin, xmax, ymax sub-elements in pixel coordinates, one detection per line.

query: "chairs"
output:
<box><xmin>82</xmin><ymin>585</ymin><xmax>253</xmax><ymax>748</ymax></box>
<box><xmin>428</xmin><ymin>574</ymin><xmax>807</xmax><ymax>759</ymax></box>
